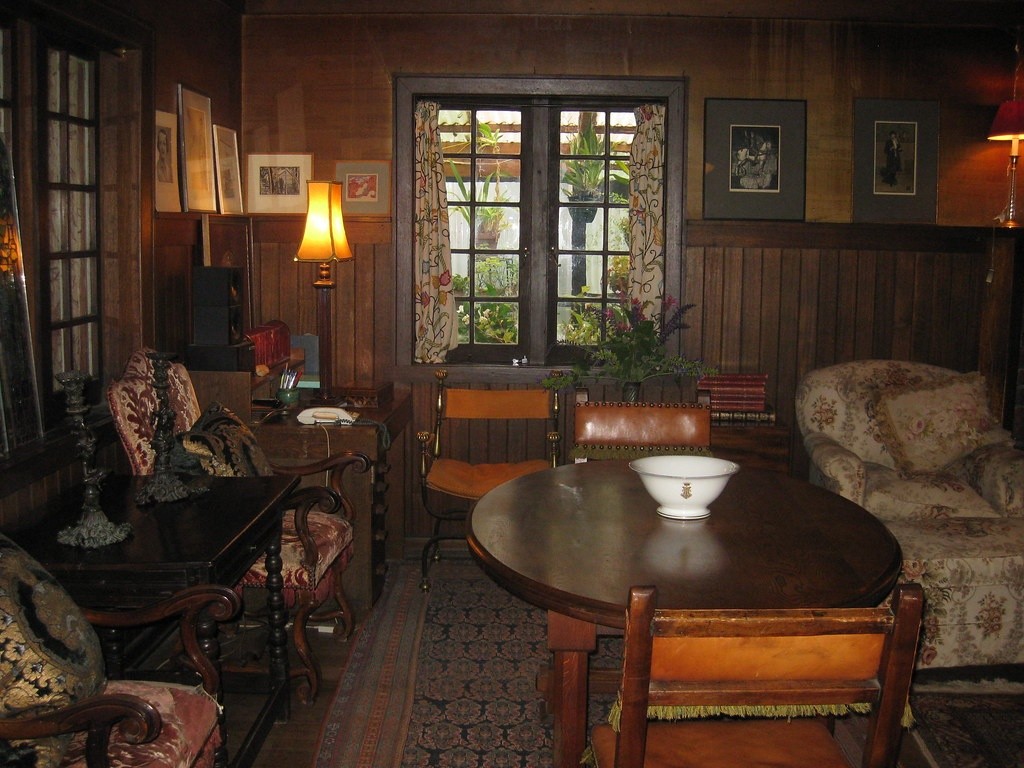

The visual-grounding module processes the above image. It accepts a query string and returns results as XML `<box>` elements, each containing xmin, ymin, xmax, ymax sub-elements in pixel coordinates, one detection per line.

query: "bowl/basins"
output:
<box><xmin>629</xmin><ymin>454</ymin><xmax>742</xmax><ymax>520</ymax></box>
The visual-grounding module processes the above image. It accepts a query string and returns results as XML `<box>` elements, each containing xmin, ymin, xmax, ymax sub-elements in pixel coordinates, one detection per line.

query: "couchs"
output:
<box><xmin>796</xmin><ymin>361</ymin><xmax>1024</xmax><ymax>694</ymax></box>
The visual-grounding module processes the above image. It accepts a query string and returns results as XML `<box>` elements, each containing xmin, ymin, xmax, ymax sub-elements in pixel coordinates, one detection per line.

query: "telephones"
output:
<box><xmin>297</xmin><ymin>406</ymin><xmax>360</xmax><ymax>426</ymax></box>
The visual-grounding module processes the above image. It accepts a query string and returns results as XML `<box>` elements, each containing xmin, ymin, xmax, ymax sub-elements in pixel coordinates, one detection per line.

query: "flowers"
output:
<box><xmin>566</xmin><ymin>280</ymin><xmax>721</xmax><ymax>384</ymax></box>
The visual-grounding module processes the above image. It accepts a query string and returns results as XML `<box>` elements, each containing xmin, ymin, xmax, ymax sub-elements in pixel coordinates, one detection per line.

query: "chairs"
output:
<box><xmin>572</xmin><ymin>389</ymin><xmax>711</xmax><ymax>459</ymax></box>
<box><xmin>691</xmin><ymin>372</ymin><xmax>795</xmax><ymax>476</ymax></box>
<box><xmin>104</xmin><ymin>347</ymin><xmax>370</xmax><ymax>693</ymax></box>
<box><xmin>589</xmin><ymin>581</ymin><xmax>927</xmax><ymax>768</ymax></box>
<box><xmin>418</xmin><ymin>369</ymin><xmax>563</xmax><ymax>592</ymax></box>
<box><xmin>1</xmin><ymin>586</ymin><xmax>243</xmax><ymax>767</ymax></box>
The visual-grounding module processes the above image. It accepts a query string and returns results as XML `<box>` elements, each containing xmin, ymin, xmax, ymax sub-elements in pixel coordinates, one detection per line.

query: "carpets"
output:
<box><xmin>314</xmin><ymin>558</ymin><xmax>933</xmax><ymax>767</ymax></box>
<box><xmin>902</xmin><ymin>676</ymin><xmax>1024</xmax><ymax>768</ymax></box>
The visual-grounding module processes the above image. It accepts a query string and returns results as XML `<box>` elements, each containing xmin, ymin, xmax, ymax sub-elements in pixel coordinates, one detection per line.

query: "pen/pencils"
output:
<box><xmin>279</xmin><ymin>368</ymin><xmax>302</xmax><ymax>389</ymax></box>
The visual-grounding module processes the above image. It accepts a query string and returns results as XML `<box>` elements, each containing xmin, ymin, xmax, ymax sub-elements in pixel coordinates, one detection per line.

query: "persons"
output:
<box><xmin>884</xmin><ymin>131</ymin><xmax>902</xmax><ymax>187</ymax></box>
<box><xmin>156</xmin><ymin>129</ymin><xmax>173</xmax><ymax>183</ymax></box>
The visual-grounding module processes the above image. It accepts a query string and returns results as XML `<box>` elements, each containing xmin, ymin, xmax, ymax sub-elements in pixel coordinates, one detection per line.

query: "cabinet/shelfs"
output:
<box><xmin>171</xmin><ymin>349</ymin><xmax>416</xmax><ymax>616</ymax></box>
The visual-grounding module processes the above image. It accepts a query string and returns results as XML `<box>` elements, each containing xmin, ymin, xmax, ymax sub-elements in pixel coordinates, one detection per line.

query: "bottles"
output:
<box><xmin>276</xmin><ymin>388</ymin><xmax>299</xmax><ymax>409</ymax></box>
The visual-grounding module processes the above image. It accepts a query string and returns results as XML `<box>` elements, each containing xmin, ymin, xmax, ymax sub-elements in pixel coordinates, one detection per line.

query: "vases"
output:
<box><xmin>623</xmin><ymin>381</ymin><xmax>641</xmax><ymax>404</ymax></box>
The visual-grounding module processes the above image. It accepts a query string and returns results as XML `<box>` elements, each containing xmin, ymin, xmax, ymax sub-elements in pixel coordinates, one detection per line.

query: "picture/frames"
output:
<box><xmin>701</xmin><ymin>98</ymin><xmax>806</xmax><ymax>222</ymax></box>
<box><xmin>850</xmin><ymin>97</ymin><xmax>941</xmax><ymax>224</ymax></box>
<box><xmin>148</xmin><ymin>86</ymin><xmax>392</xmax><ymax>332</ymax></box>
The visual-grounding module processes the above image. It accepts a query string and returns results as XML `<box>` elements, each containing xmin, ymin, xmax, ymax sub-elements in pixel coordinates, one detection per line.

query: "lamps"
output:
<box><xmin>293</xmin><ymin>180</ymin><xmax>353</xmax><ymax>405</ymax></box>
<box><xmin>988</xmin><ymin>99</ymin><xmax>1024</xmax><ymax>230</ymax></box>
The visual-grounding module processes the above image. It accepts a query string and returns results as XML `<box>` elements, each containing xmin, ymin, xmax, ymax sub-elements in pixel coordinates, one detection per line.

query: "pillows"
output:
<box><xmin>180</xmin><ymin>403</ymin><xmax>273</xmax><ymax>477</ymax></box>
<box><xmin>866</xmin><ymin>374</ymin><xmax>1017</xmax><ymax>486</ymax></box>
<box><xmin>1</xmin><ymin>535</ymin><xmax>107</xmax><ymax>768</ymax></box>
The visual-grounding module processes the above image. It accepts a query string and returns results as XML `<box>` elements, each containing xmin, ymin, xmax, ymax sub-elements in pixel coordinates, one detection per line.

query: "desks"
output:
<box><xmin>465</xmin><ymin>459</ymin><xmax>904</xmax><ymax>768</ymax></box>
<box><xmin>12</xmin><ymin>474</ymin><xmax>304</xmax><ymax>768</ymax></box>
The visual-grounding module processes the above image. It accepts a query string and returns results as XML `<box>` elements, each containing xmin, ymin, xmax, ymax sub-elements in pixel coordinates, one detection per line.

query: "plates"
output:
<box><xmin>297</xmin><ymin>406</ymin><xmax>354</xmax><ymax>424</ymax></box>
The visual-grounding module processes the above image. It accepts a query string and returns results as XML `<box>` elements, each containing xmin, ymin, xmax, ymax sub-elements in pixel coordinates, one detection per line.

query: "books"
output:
<box><xmin>696</xmin><ymin>374</ymin><xmax>776</xmax><ymax>422</ymax></box>
